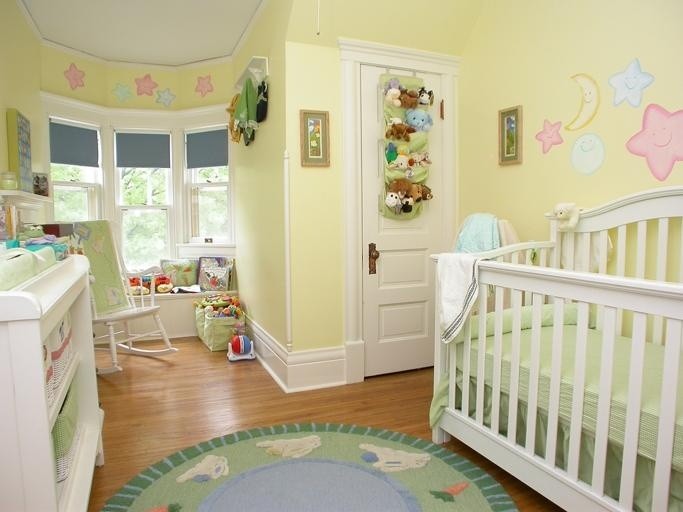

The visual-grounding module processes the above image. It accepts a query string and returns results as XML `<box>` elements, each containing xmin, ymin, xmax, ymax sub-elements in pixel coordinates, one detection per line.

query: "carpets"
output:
<box><xmin>100</xmin><ymin>421</ymin><xmax>520</xmax><ymax>511</ymax></box>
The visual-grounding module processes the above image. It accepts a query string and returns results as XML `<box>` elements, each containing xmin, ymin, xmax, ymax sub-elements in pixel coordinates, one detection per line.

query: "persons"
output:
<box><xmin>34</xmin><ymin>176</ymin><xmax>48</xmax><ymax>195</ymax></box>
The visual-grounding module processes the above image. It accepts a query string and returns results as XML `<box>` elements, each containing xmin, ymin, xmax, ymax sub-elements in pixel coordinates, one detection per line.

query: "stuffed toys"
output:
<box><xmin>18</xmin><ymin>225</ymin><xmax>45</xmax><ymax>239</ymax></box>
<box><xmin>194</xmin><ymin>294</ymin><xmax>242</xmax><ymax>318</ymax></box>
<box><xmin>544</xmin><ymin>202</ymin><xmax>613</xmax><ymax>272</ymax></box>
<box><xmin>383</xmin><ymin>78</ymin><xmax>434</xmax><ymax>212</ymax></box>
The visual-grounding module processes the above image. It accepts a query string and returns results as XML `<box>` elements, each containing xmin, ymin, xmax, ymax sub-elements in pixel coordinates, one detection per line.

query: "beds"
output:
<box><xmin>428</xmin><ymin>184</ymin><xmax>682</xmax><ymax>511</ymax></box>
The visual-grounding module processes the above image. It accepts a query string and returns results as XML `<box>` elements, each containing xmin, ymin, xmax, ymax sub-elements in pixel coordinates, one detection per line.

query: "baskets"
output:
<box><xmin>43</xmin><ymin>309</ymin><xmax>83</xmax><ymax>482</ymax></box>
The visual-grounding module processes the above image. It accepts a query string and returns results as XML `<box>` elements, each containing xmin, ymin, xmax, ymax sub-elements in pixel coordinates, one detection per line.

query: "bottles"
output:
<box><xmin>0</xmin><ymin>171</ymin><xmax>17</xmax><ymax>189</ymax></box>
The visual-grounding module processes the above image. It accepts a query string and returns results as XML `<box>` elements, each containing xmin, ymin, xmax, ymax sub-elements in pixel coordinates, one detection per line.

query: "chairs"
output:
<box><xmin>72</xmin><ymin>217</ymin><xmax>179</xmax><ymax>376</ymax></box>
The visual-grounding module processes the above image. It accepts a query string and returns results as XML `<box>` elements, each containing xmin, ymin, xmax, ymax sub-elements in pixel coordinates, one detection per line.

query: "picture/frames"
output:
<box><xmin>497</xmin><ymin>105</ymin><xmax>523</xmax><ymax>168</ymax></box>
<box><xmin>299</xmin><ymin>110</ymin><xmax>331</xmax><ymax>168</ymax></box>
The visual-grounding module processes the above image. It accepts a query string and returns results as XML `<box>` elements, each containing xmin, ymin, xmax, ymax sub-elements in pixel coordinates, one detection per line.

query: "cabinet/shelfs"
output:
<box><xmin>0</xmin><ymin>251</ymin><xmax>106</xmax><ymax>512</ymax></box>
